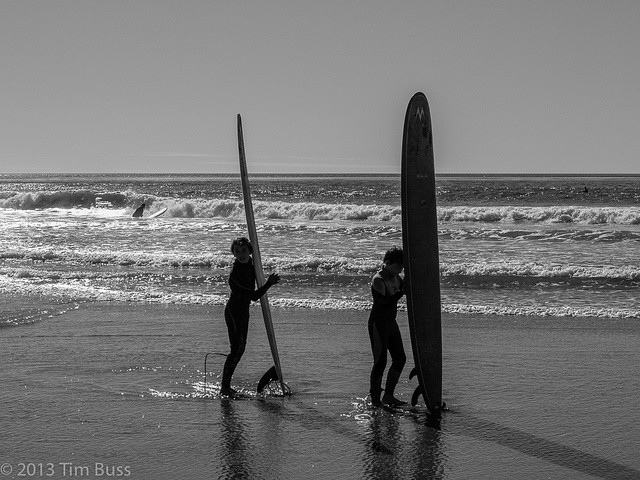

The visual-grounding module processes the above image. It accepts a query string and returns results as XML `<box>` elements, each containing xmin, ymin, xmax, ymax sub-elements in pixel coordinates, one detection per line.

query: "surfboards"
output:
<box><xmin>236</xmin><ymin>112</ymin><xmax>291</xmax><ymax>397</ymax></box>
<box><xmin>400</xmin><ymin>91</ymin><xmax>444</xmax><ymax>416</ymax></box>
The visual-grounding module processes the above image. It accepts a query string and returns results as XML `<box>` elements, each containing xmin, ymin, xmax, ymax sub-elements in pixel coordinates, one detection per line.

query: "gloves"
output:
<box><xmin>246</xmin><ymin>273</ymin><xmax>280</xmax><ymax>302</ymax></box>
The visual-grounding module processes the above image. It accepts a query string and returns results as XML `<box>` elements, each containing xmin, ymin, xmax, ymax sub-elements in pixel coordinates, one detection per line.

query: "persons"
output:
<box><xmin>132</xmin><ymin>203</ymin><xmax>145</xmax><ymax>217</ymax></box>
<box><xmin>367</xmin><ymin>247</ymin><xmax>413</xmax><ymax>411</ymax></box>
<box><xmin>219</xmin><ymin>237</ymin><xmax>281</xmax><ymax>398</ymax></box>
